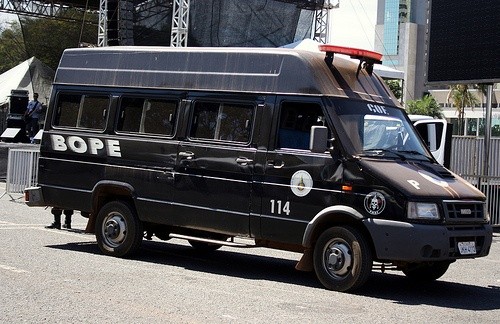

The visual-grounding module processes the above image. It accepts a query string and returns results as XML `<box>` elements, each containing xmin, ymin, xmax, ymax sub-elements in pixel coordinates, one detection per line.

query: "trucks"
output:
<box><xmin>353</xmin><ymin>112</ymin><xmax>448</xmax><ymax>180</ymax></box>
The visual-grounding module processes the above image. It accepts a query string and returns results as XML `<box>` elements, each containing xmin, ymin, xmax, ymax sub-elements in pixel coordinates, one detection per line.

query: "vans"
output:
<box><xmin>23</xmin><ymin>43</ymin><xmax>493</xmax><ymax>293</ymax></box>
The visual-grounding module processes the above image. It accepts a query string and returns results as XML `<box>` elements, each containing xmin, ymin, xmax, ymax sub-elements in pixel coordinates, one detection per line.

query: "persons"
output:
<box><xmin>46</xmin><ymin>208</ymin><xmax>74</xmax><ymax>230</ymax></box>
<box><xmin>24</xmin><ymin>93</ymin><xmax>43</xmax><ymax>137</ymax></box>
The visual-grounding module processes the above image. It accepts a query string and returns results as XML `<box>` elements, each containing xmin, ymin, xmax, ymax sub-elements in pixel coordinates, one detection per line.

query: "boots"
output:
<box><xmin>62</xmin><ymin>215</ymin><xmax>71</xmax><ymax>229</ymax></box>
<box><xmin>44</xmin><ymin>215</ymin><xmax>61</xmax><ymax>230</ymax></box>
